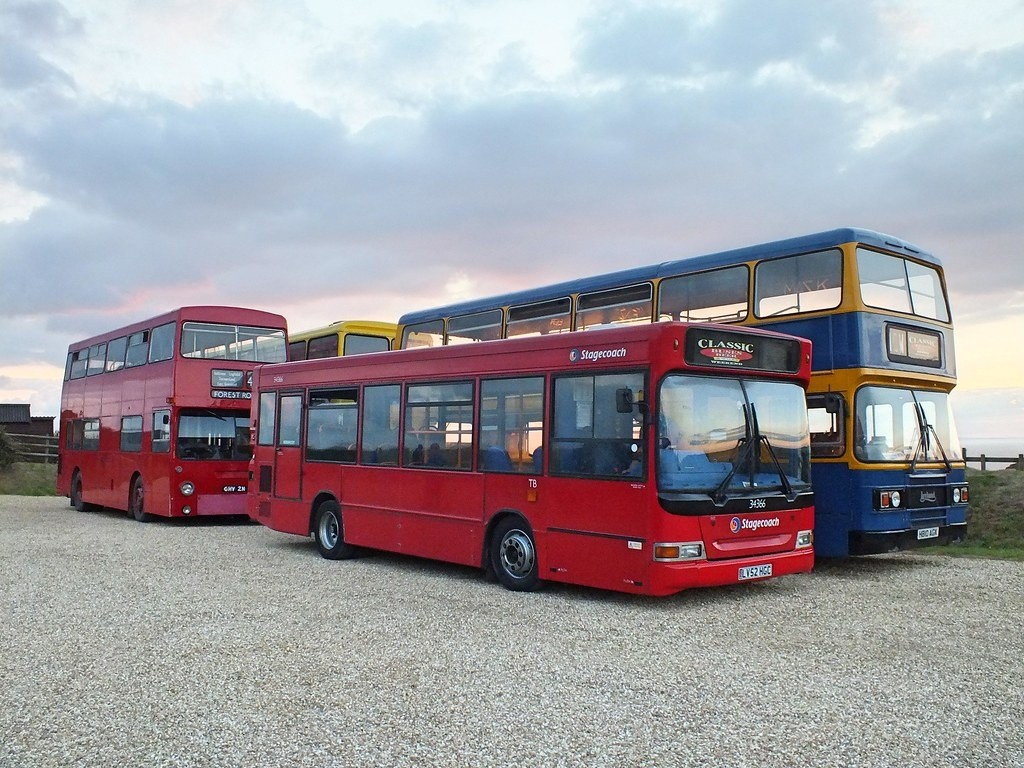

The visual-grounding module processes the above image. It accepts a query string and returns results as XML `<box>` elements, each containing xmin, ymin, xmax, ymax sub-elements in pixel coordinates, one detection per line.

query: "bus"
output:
<box><xmin>200</xmin><ymin>321</ymin><xmax>439</xmax><ymax>434</ymax></box>
<box><xmin>58</xmin><ymin>306</ymin><xmax>291</xmax><ymax>522</ymax></box>
<box><xmin>393</xmin><ymin>227</ymin><xmax>970</xmax><ymax>556</ymax></box>
<box><xmin>247</xmin><ymin>320</ymin><xmax>815</xmax><ymax>597</ymax></box>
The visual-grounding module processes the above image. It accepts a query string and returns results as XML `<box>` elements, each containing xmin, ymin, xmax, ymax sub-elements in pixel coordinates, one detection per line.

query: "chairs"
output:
<box><xmin>482</xmin><ymin>446</ymin><xmax>514</xmax><ymax>471</ymax></box>
<box><xmin>375</xmin><ymin>443</ymin><xmax>409</xmax><ymax>465</ymax></box>
<box><xmin>349</xmin><ymin>442</ymin><xmax>375</xmax><ymax>463</ymax></box>
<box><xmin>532</xmin><ymin>444</ymin><xmax>577</xmax><ymax>473</ymax></box>
<box><xmin>418</xmin><ymin>425</ymin><xmax>444</xmax><ymax>446</ymax></box>
<box><xmin>319</xmin><ymin>423</ymin><xmax>348</xmax><ymax>461</ymax></box>
